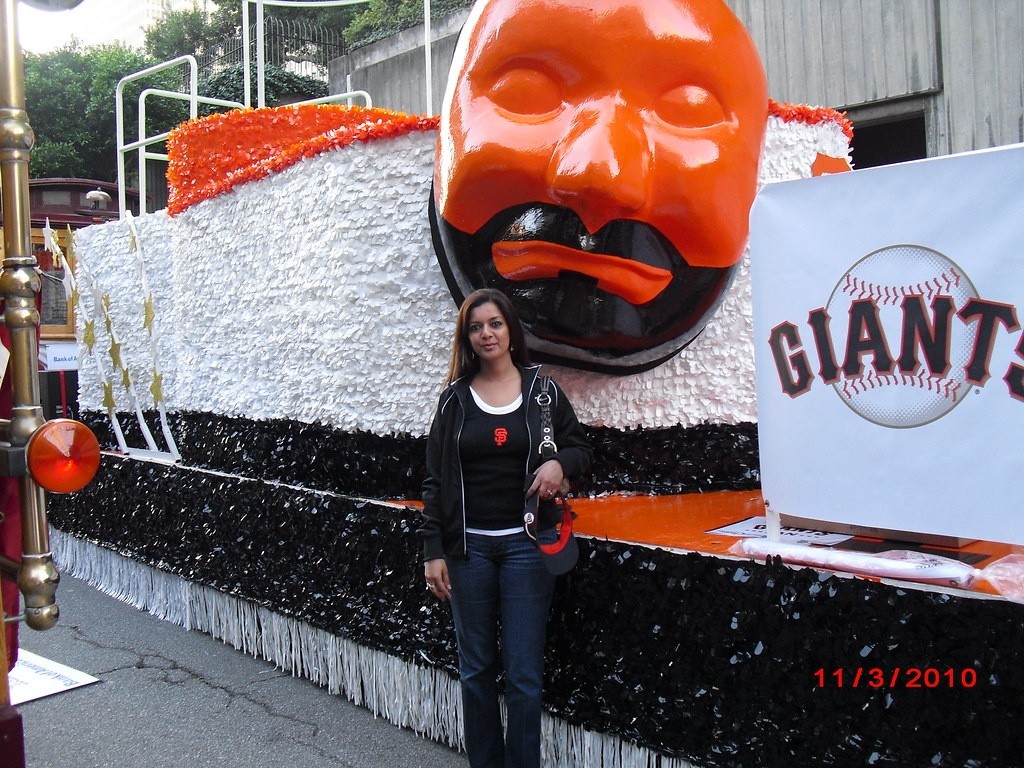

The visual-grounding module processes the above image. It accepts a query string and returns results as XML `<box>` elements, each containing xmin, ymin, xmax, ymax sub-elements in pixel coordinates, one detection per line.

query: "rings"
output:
<box><xmin>426</xmin><ymin>583</ymin><xmax>434</xmax><ymax>590</ymax></box>
<box><xmin>547</xmin><ymin>490</ymin><xmax>552</xmax><ymax>495</ymax></box>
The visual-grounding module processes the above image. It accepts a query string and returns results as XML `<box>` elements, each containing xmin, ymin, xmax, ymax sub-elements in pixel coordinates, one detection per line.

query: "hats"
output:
<box><xmin>523</xmin><ymin>490</ymin><xmax>579</xmax><ymax>575</ymax></box>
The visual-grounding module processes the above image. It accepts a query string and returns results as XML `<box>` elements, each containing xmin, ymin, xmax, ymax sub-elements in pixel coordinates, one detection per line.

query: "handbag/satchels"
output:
<box><xmin>522</xmin><ymin>376</ymin><xmax>558</xmax><ymax>492</ymax></box>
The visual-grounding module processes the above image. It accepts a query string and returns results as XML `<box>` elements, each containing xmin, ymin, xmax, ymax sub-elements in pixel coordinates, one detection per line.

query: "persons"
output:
<box><xmin>421</xmin><ymin>287</ymin><xmax>593</xmax><ymax>768</ymax></box>
